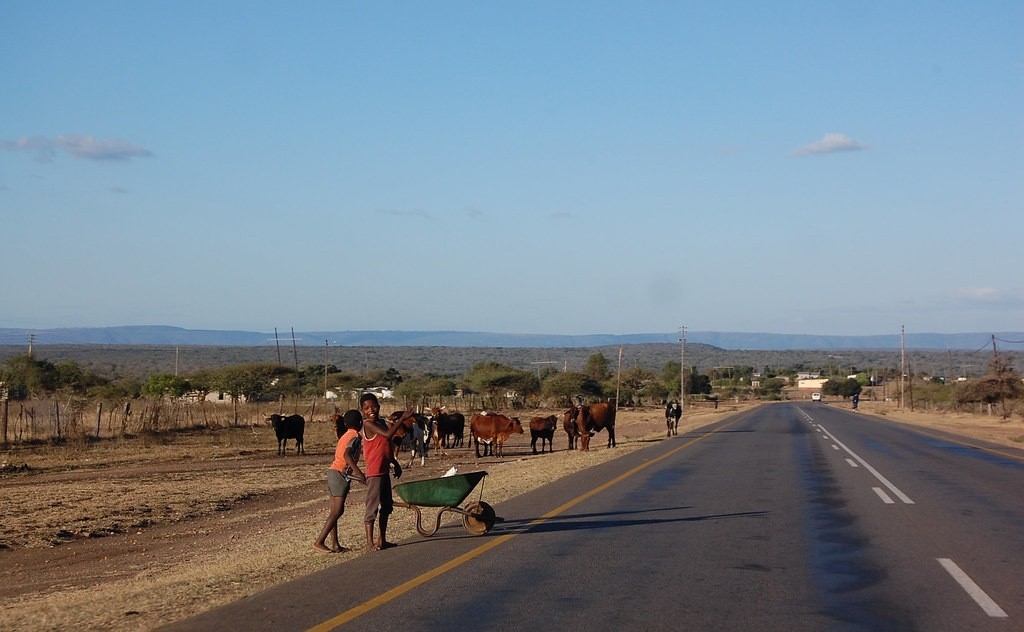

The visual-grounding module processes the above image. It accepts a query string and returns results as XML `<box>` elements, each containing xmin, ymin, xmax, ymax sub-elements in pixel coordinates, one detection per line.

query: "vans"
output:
<box><xmin>812</xmin><ymin>393</ymin><xmax>821</xmax><ymax>402</ymax></box>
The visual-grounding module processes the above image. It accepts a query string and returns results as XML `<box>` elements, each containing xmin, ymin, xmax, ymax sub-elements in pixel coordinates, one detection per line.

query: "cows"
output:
<box><xmin>385</xmin><ymin>406</ymin><xmax>465</xmax><ymax>468</ymax></box>
<box><xmin>330</xmin><ymin>408</ymin><xmax>364</xmax><ymax>440</ymax></box>
<box><xmin>529</xmin><ymin>414</ymin><xmax>558</xmax><ymax>455</ymax></box>
<box><xmin>467</xmin><ymin>410</ymin><xmax>525</xmax><ymax>459</ymax></box>
<box><xmin>563</xmin><ymin>402</ymin><xmax>616</xmax><ymax>451</ymax></box>
<box><xmin>665</xmin><ymin>400</ymin><xmax>683</xmax><ymax>437</ymax></box>
<box><xmin>265</xmin><ymin>413</ymin><xmax>305</xmax><ymax>455</ymax></box>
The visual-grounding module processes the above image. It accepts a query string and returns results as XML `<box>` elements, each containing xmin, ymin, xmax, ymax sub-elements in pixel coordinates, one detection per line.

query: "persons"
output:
<box><xmin>360</xmin><ymin>393</ymin><xmax>416</xmax><ymax>553</ymax></box>
<box><xmin>313</xmin><ymin>409</ymin><xmax>366</xmax><ymax>553</ymax></box>
<box><xmin>853</xmin><ymin>393</ymin><xmax>858</xmax><ymax>413</ymax></box>
<box><xmin>714</xmin><ymin>396</ymin><xmax>718</xmax><ymax>409</ymax></box>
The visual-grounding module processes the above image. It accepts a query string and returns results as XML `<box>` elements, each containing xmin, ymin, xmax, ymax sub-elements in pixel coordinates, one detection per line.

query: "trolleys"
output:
<box><xmin>345</xmin><ymin>469</ymin><xmax>506</xmax><ymax>539</ymax></box>
